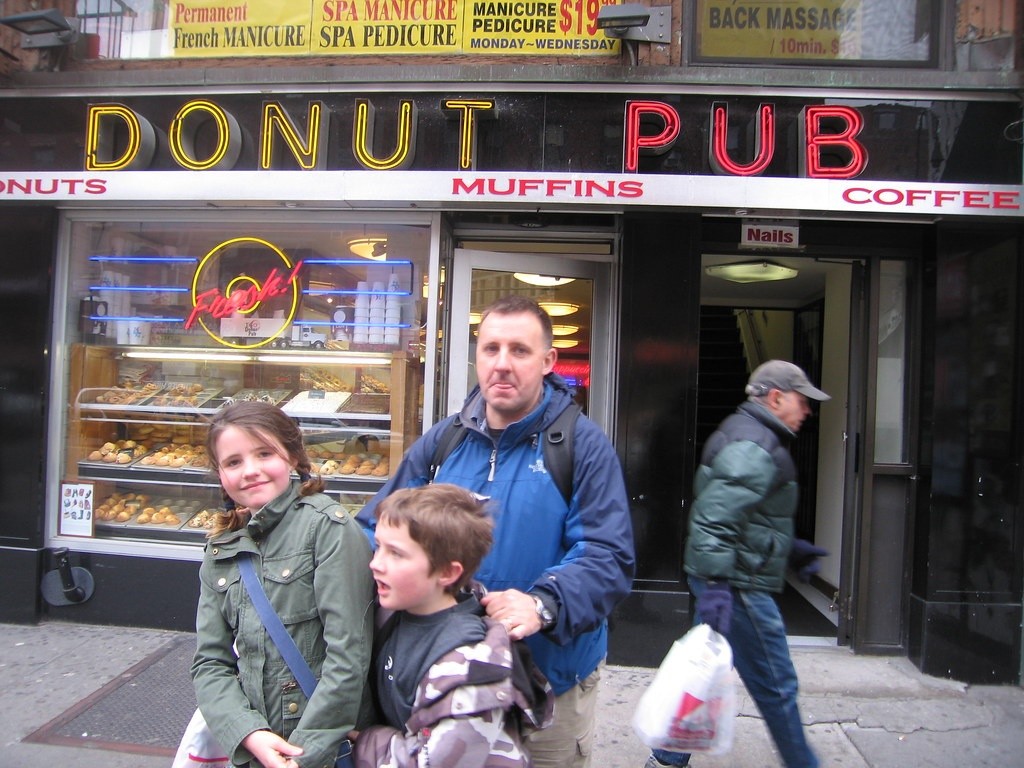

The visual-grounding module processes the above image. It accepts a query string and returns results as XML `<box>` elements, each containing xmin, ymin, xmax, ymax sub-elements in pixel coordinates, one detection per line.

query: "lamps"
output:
<box><xmin>1</xmin><ymin>8</ymin><xmax>80</xmax><ymax>43</ymax></box>
<box><xmin>595</xmin><ymin>4</ymin><xmax>652</xmax><ymax>33</ymax></box>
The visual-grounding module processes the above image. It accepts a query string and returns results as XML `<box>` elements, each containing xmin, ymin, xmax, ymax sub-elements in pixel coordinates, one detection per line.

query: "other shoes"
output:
<box><xmin>644</xmin><ymin>755</ymin><xmax>693</xmax><ymax>768</ymax></box>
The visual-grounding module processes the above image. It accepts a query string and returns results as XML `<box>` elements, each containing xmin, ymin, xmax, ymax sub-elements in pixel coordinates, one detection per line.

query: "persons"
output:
<box><xmin>191</xmin><ymin>400</ymin><xmax>375</xmax><ymax>768</ymax></box>
<box><xmin>352</xmin><ymin>296</ymin><xmax>636</xmax><ymax>768</ymax></box>
<box><xmin>644</xmin><ymin>359</ymin><xmax>832</xmax><ymax>768</ymax></box>
<box><xmin>347</xmin><ymin>483</ymin><xmax>557</xmax><ymax>768</ymax></box>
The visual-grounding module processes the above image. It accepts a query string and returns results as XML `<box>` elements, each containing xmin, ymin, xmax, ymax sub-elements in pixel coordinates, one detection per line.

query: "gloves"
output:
<box><xmin>788</xmin><ymin>538</ymin><xmax>832</xmax><ymax>584</ymax></box>
<box><xmin>694</xmin><ymin>581</ymin><xmax>734</xmax><ymax>635</ymax></box>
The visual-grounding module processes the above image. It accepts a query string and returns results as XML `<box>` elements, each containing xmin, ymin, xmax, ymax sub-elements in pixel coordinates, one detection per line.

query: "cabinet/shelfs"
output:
<box><xmin>69</xmin><ymin>341</ymin><xmax>413</xmax><ymax>543</ymax></box>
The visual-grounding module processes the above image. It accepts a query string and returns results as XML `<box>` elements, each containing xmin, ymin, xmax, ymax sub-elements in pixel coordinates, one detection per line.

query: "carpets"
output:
<box><xmin>21</xmin><ymin>631</ymin><xmax>207</xmax><ymax>757</ymax></box>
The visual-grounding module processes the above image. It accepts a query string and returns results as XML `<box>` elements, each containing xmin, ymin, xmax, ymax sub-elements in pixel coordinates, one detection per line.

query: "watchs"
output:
<box><xmin>529</xmin><ymin>593</ymin><xmax>554</xmax><ymax>630</ymax></box>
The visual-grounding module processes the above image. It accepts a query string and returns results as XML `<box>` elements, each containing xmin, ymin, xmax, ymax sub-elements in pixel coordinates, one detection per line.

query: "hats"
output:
<box><xmin>748</xmin><ymin>360</ymin><xmax>832</xmax><ymax>402</ymax></box>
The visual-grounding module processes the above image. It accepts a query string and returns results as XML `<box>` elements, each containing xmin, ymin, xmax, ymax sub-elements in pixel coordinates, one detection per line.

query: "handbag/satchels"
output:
<box><xmin>172</xmin><ymin>708</ymin><xmax>229</xmax><ymax>768</ymax></box>
<box><xmin>632</xmin><ymin>624</ymin><xmax>736</xmax><ymax>756</ymax></box>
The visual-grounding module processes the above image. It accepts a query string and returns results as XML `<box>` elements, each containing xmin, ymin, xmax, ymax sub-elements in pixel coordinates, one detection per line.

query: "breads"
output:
<box><xmin>298</xmin><ymin>367</ymin><xmax>390</xmax><ymax>393</ymax></box>
<box><xmin>304</xmin><ymin>433</ymin><xmax>390</xmax><ymax>477</ymax></box>
<box><xmin>86</xmin><ymin>425</ymin><xmax>221</xmax><ymax>528</ymax></box>
<box><xmin>95</xmin><ymin>380</ymin><xmax>274</xmax><ymax>409</ymax></box>
